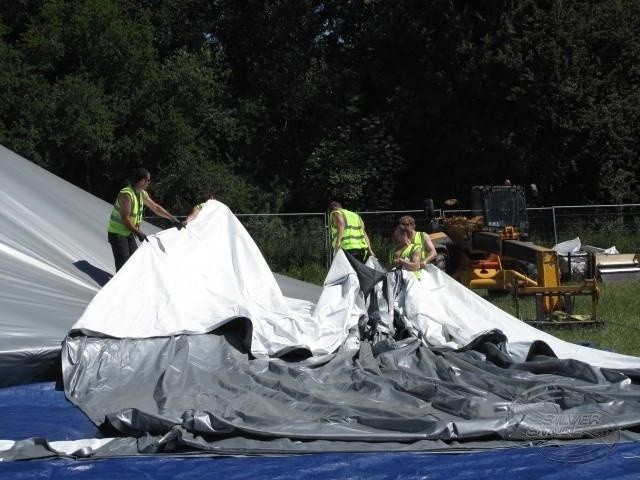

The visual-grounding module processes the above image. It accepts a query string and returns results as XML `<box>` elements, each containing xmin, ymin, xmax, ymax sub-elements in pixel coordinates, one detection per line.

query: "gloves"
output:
<box><xmin>136</xmin><ymin>231</ymin><xmax>149</xmax><ymax>242</ymax></box>
<box><xmin>172</xmin><ymin>217</ymin><xmax>186</xmax><ymax>230</ymax></box>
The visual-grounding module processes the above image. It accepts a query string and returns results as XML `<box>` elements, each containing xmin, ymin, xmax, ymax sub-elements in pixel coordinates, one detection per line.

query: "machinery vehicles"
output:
<box><xmin>422</xmin><ymin>182</ymin><xmax>604</xmax><ymax>329</ymax></box>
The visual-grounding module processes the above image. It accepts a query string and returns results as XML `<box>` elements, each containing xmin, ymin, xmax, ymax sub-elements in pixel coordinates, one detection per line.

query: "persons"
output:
<box><xmin>107</xmin><ymin>167</ymin><xmax>187</xmax><ymax>274</ymax></box>
<box><xmin>182</xmin><ymin>191</ymin><xmax>217</xmax><ymax>225</ymax></box>
<box><xmin>326</xmin><ymin>200</ymin><xmax>372</xmax><ymax>267</ymax></box>
<box><xmin>384</xmin><ymin>215</ymin><xmax>438</xmax><ymax>269</ymax></box>
<box><xmin>388</xmin><ymin>223</ymin><xmax>422</xmax><ymax>272</ymax></box>
